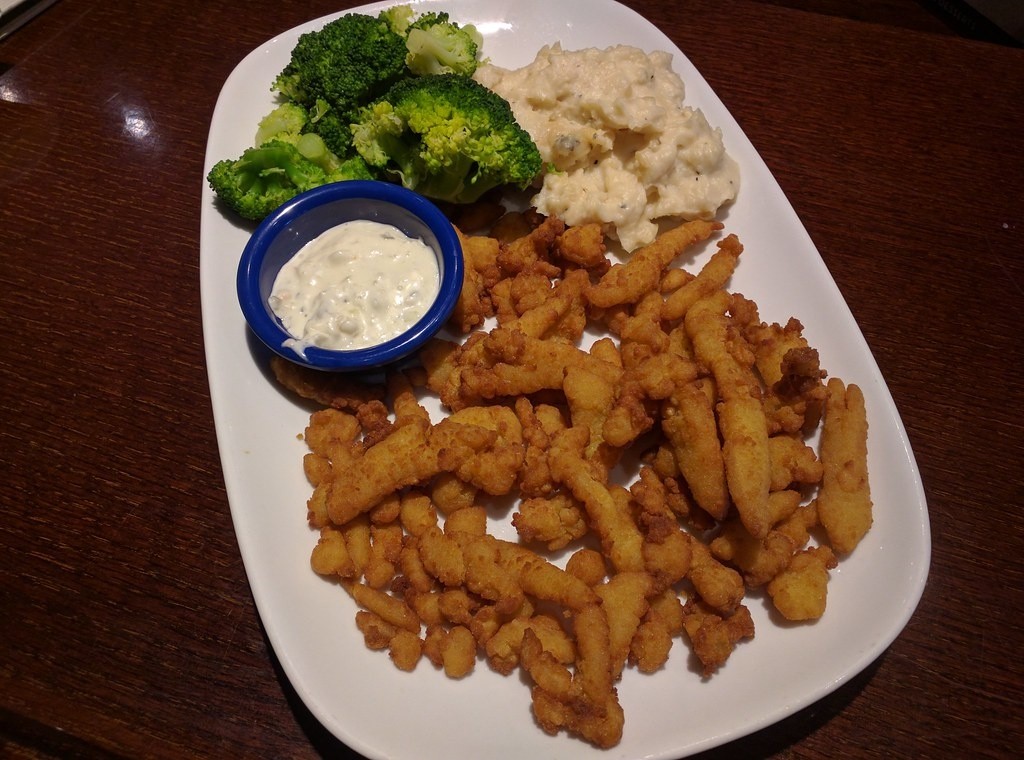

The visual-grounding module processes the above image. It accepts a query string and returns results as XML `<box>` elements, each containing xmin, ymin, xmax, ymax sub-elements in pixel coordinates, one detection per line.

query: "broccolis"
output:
<box><xmin>206</xmin><ymin>5</ymin><xmax>541</xmax><ymax>220</ymax></box>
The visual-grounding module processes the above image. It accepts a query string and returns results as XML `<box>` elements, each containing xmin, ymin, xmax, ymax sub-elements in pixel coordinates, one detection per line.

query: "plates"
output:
<box><xmin>198</xmin><ymin>0</ymin><xmax>933</xmax><ymax>760</ymax></box>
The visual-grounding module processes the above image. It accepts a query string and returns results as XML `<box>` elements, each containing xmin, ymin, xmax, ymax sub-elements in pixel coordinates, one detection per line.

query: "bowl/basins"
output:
<box><xmin>236</xmin><ymin>180</ymin><xmax>463</xmax><ymax>372</ymax></box>
